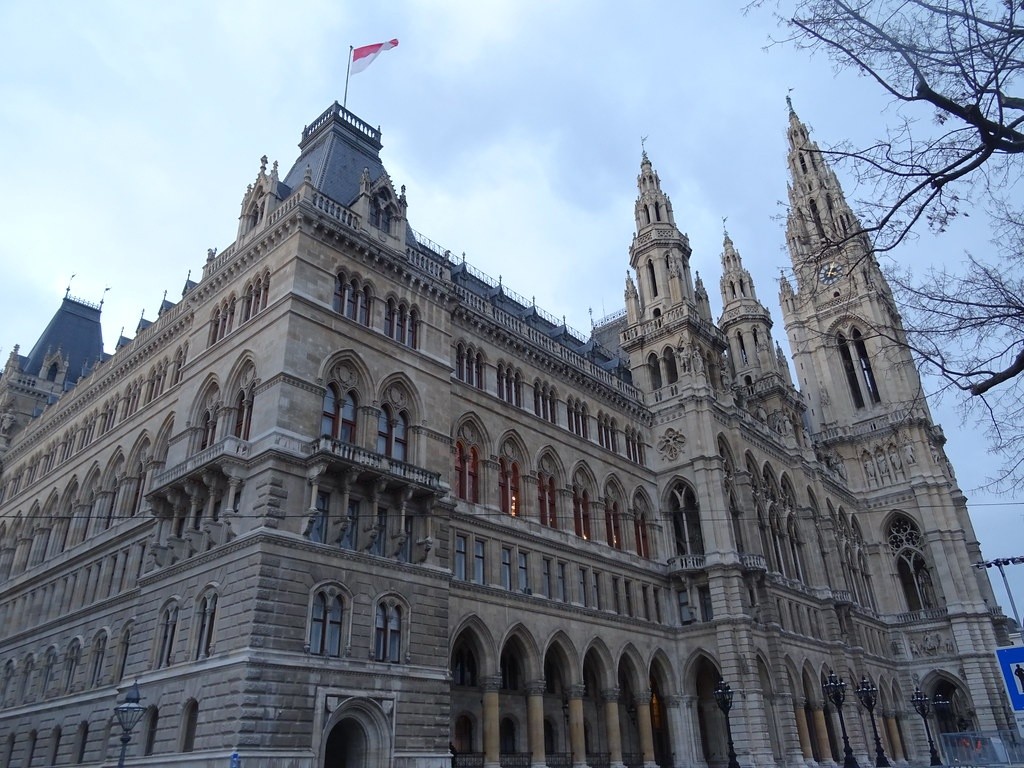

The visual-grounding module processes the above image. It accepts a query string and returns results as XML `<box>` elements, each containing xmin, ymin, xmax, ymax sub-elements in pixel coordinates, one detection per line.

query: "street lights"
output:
<box><xmin>712</xmin><ymin>676</ymin><xmax>740</xmax><ymax>768</ymax></box>
<box><xmin>909</xmin><ymin>684</ymin><xmax>944</xmax><ymax>766</ymax></box>
<box><xmin>823</xmin><ymin>666</ymin><xmax>860</xmax><ymax>768</ymax></box>
<box><xmin>854</xmin><ymin>674</ymin><xmax>890</xmax><ymax>767</ymax></box>
<box><xmin>113</xmin><ymin>678</ymin><xmax>149</xmax><ymax>768</ymax></box>
<box><xmin>976</xmin><ymin>555</ymin><xmax>1024</xmax><ymax>629</ymax></box>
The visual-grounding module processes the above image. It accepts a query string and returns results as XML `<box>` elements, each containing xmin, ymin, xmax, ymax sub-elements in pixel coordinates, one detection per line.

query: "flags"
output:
<box><xmin>349</xmin><ymin>38</ymin><xmax>399</xmax><ymax>75</ymax></box>
